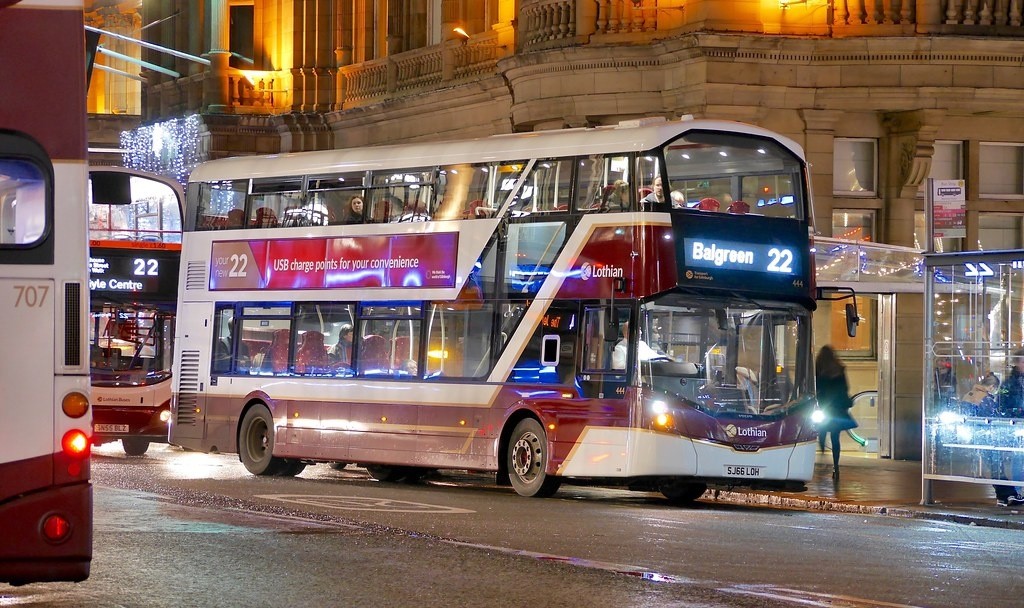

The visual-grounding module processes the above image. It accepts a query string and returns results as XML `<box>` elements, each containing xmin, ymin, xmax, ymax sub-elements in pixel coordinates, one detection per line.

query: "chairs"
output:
<box><xmin>217</xmin><ymin>329</ymin><xmax>420</xmax><ymax>377</ymax></box>
<box><xmin>225</xmin><ymin>187</ymin><xmax>752</xmax><ymax>230</ymax></box>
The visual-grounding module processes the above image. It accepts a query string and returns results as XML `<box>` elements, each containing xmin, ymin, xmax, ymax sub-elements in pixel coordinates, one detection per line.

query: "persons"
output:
<box><xmin>642</xmin><ymin>176</ymin><xmax>679</xmax><ymax>208</ymax></box>
<box><xmin>218</xmin><ymin>317</ymin><xmax>250</xmax><ymax>372</ymax></box>
<box><xmin>652</xmin><ymin>327</ymin><xmax>674</xmax><ymax>360</ymax></box>
<box><xmin>611</xmin><ymin>322</ymin><xmax>665</xmax><ymax>370</ymax></box>
<box><xmin>936</xmin><ymin>356</ymin><xmax>957</xmax><ymax>464</ymax></box>
<box><xmin>1003</xmin><ymin>349</ymin><xmax>1024</xmax><ymax>493</ymax></box>
<box><xmin>327</xmin><ymin>324</ymin><xmax>353</xmax><ymax>364</ymax></box>
<box><xmin>401</xmin><ymin>359</ymin><xmax>418</xmax><ymax>376</ymax></box>
<box><xmin>344</xmin><ymin>194</ymin><xmax>372</xmax><ymax>224</ymax></box>
<box><xmin>815</xmin><ymin>345</ymin><xmax>857</xmax><ymax>478</ymax></box>
<box><xmin>959</xmin><ymin>373</ymin><xmax>1024</xmax><ymax>506</ymax></box>
<box><xmin>608</xmin><ymin>180</ymin><xmax>629</xmax><ymax>204</ymax></box>
<box><xmin>670</xmin><ymin>191</ymin><xmax>684</xmax><ymax>205</ymax></box>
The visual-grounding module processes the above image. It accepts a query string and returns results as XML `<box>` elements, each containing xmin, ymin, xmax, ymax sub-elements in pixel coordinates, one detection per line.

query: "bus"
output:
<box><xmin>165</xmin><ymin>114</ymin><xmax>860</xmax><ymax>498</ymax></box>
<box><xmin>85</xmin><ymin>164</ymin><xmax>185</xmax><ymax>458</ymax></box>
<box><xmin>0</xmin><ymin>0</ymin><xmax>94</xmax><ymax>588</ymax></box>
